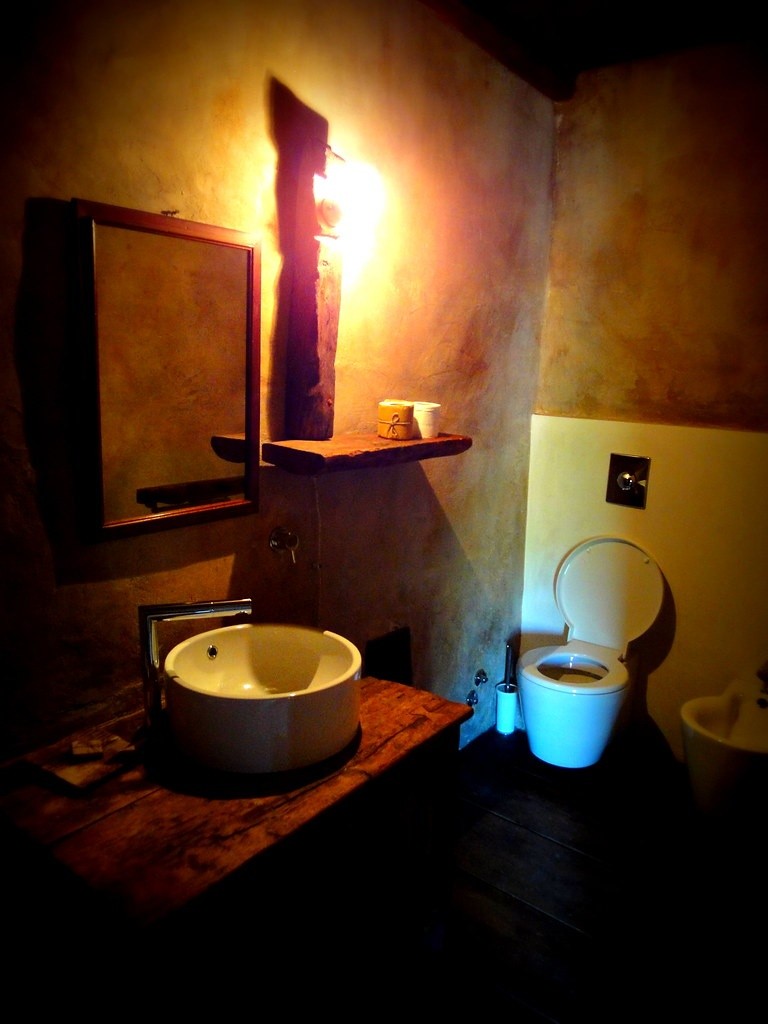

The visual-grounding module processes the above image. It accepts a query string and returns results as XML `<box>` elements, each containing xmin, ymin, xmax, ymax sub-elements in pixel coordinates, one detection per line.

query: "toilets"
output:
<box><xmin>521</xmin><ymin>535</ymin><xmax>663</xmax><ymax>770</ymax></box>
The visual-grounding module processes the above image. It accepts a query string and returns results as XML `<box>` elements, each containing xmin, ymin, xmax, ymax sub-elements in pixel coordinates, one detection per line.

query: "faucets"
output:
<box><xmin>143</xmin><ymin>598</ymin><xmax>253</xmax><ymax>722</ymax></box>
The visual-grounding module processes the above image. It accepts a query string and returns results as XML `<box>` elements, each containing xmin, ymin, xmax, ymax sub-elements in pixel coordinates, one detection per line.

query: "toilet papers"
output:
<box><xmin>411</xmin><ymin>402</ymin><xmax>442</xmax><ymax>440</ymax></box>
<box><xmin>376</xmin><ymin>400</ymin><xmax>415</xmax><ymax>439</ymax></box>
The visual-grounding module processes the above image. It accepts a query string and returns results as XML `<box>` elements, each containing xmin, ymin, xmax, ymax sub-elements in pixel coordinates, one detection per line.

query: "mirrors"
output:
<box><xmin>61</xmin><ymin>194</ymin><xmax>263</xmax><ymax>549</ymax></box>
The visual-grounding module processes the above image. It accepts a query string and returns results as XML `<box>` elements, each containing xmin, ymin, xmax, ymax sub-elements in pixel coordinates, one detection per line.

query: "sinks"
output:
<box><xmin>682</xmin><ymin>682</ymin><xmax>768</xmax><ymax>808</ymax></box>
<box><xmin>165</xmin><ymin>624</ymin><xmax>361</xmax><ymax>771</ymax></box>
